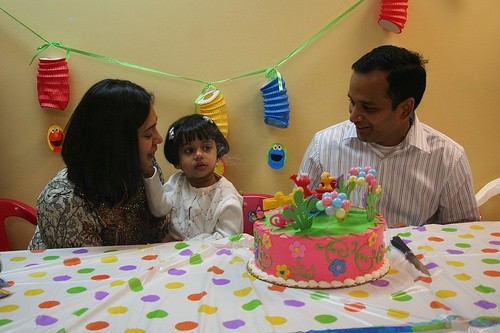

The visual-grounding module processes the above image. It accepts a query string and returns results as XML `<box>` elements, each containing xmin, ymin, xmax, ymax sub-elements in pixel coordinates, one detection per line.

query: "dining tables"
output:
<box><xmin>0</xmin><ymin>221</ymin><xmax>500</xmax><ymax>333</ymax></box>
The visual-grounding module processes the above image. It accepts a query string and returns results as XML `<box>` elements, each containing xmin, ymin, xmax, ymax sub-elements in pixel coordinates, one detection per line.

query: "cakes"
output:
<box><xmin>253</xmin><ymin>166</ymin><xmax>387</xmax><ymax>283</ymax></box>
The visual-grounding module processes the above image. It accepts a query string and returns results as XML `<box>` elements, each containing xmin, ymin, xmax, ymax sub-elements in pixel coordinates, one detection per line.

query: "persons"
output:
<box><xmin>140</xmin><ymin>114</ymin><xmax>244</xmax><ymax>242</ymax></box>
<box><xmin>25</xmin><ymin>79</ymin><xmax>171</xmax><ymax>251</ymax></box>
<box><xmin>291</xmin><ymin>45</ymin><xmax>482</xmax><ymax>229</ymax></box>
